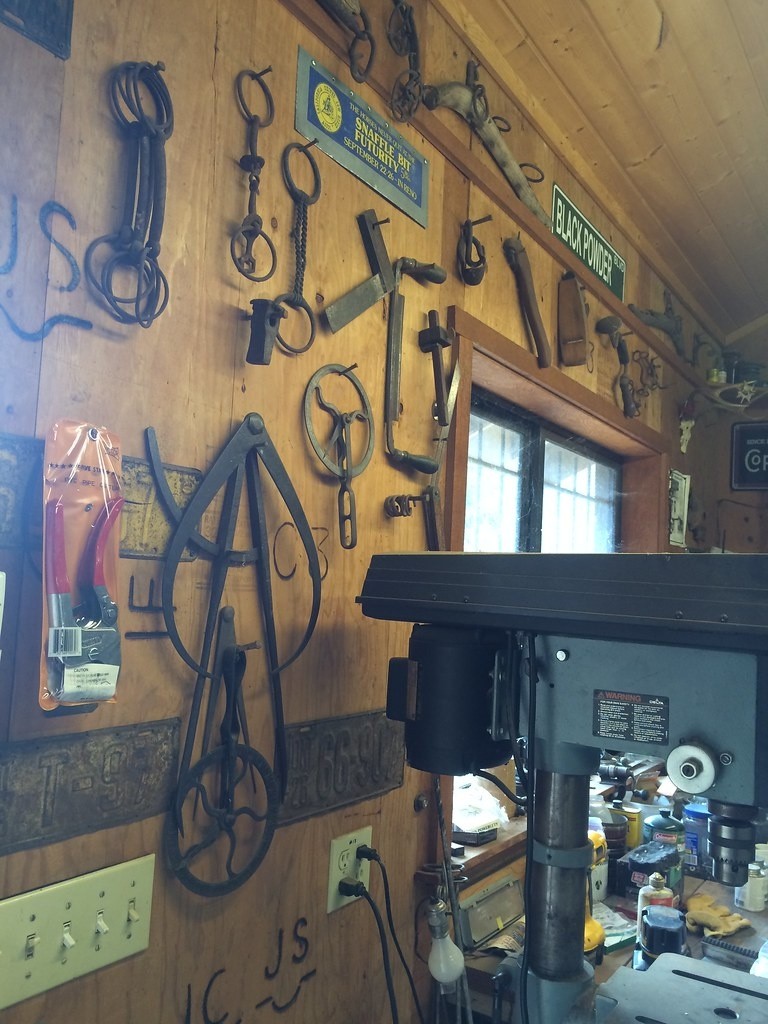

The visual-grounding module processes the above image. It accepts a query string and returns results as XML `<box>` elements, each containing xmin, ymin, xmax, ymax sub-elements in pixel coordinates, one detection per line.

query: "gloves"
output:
<box><xmin>684</xmin><ymin>894</ymin><xmax>750</xmax><ymax>939</ymax></box>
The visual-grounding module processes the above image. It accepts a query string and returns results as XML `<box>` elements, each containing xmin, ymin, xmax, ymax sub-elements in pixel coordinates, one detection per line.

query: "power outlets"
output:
<box><xmin>326</xmin><ymin>824</ymin><xmax>373</xmax><ymax>915</ymax></box>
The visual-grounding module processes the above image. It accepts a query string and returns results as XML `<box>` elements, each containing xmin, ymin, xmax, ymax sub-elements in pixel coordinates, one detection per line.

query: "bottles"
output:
<box><xmin>735</xmin><ymin>865</ymin><xmax>765</xmax><ymax>912</ymax></box>
<box><xmin>752</xmin><ymin>859</ymin><xmax>768</xmax><ymax>901</ymax></box>
<box><xmin>718</xmin><ymin>370</ymin><xmax>726</xmax><ymax>383</ymax></box>
<box><xmin>709</xmin><ymin>368</ymin><xmax>718</xmax><ymax>382</ymax></box>
<box><xmin>682</xmin><ymin>804</ymin><xmax>712</xmax><ymax>878</ymax></box>
<box><xmin>589</xmin><ymin>795</ymin><xmax>614</xmax><ymax>824</ymax></box>
<box><xmin>637</xmin><ymin>872</ymin><xmax>673</xmax><ymax>939</ymax></box>
<box><xmin>586</xmin><ymin>817</ymin><xmax>608</xmax><ymax>901</ymax></box>
<box><xmin>646</xmin><ymin>809</ymin><xmax>685</xmax><ymax>902</ymax></box>
